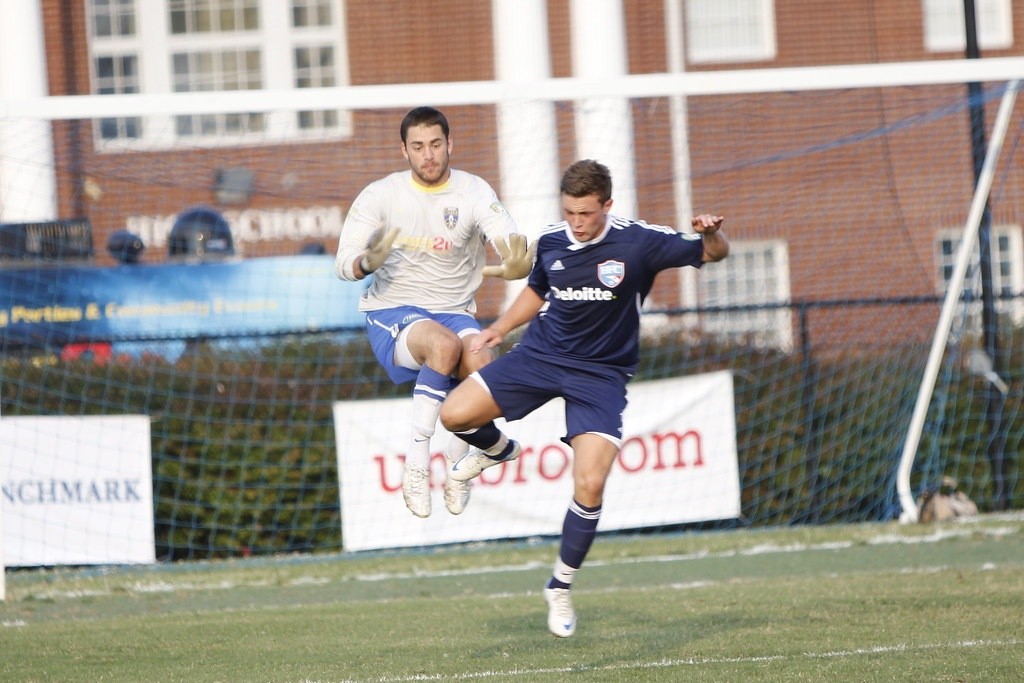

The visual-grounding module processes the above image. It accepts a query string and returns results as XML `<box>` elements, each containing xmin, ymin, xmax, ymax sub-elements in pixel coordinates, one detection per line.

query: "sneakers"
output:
<box><xmin>450</xmin><ymin>440</ymin><xmax>521</xmax><ymax>481</ymax></box>
<box><xmin>402</xmin><ymin>456</ymin><xmax>430</xmax><ymax>516</ymax></box>
<box><xmin>543</xmin><ymin>578</ymin><xmax>576</xmax><ymax>638</ymax></box>
<box><xmin>443</xmin><ymin>448</ymin><xmax>469</xmax><ymax>514</ymax></box>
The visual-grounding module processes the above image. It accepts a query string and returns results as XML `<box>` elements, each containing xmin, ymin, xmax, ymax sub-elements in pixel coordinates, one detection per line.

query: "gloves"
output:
<box><xmin>360</xmin><ymin>227</ymin><xmax>401</xmax><ymax>275</ymax></box>
<box><xmin>481</xmin><ymin>233</ymin><xmax>538</xmax><ymax>280</ymax></box>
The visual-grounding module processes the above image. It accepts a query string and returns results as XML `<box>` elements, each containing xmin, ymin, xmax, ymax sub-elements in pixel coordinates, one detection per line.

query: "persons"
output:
<box><xmin>334</xmin><ymin>105</ymin><xmax>545</xmax><ymax>526</ymax></box>
<box><xmin>437</xmin><ymin>156</ymin><xmax>731</xmax><ymax>639</ymax></box>
